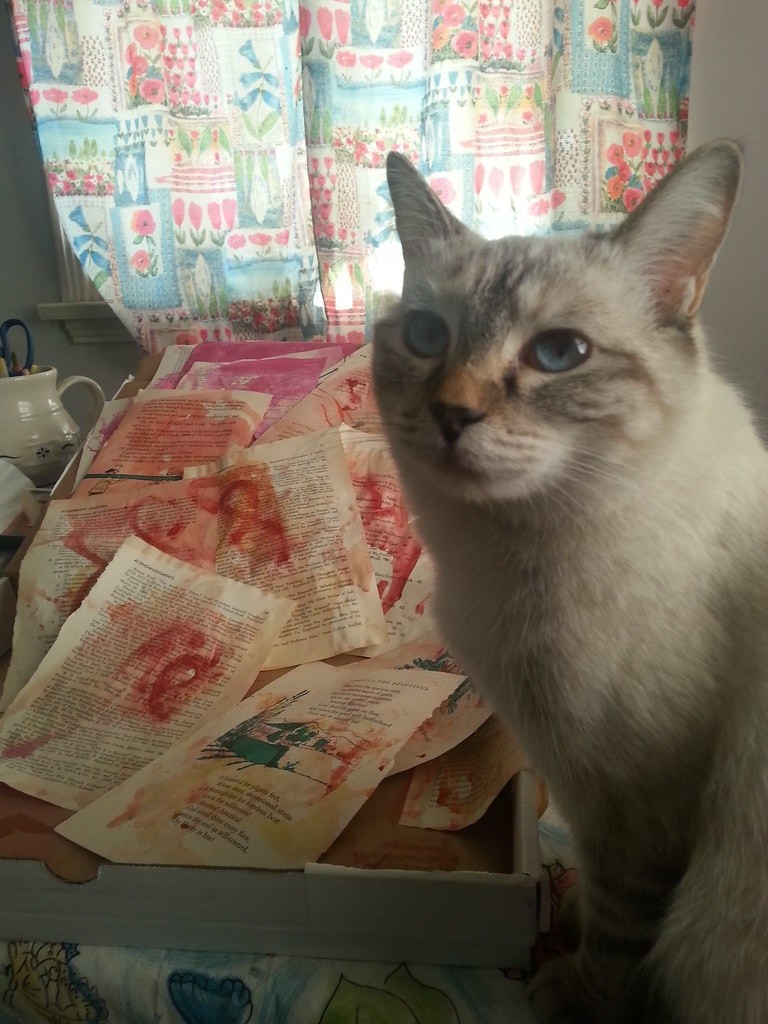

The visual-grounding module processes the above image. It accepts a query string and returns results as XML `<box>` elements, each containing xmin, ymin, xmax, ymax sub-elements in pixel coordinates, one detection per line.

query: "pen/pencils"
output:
<box><xmin>0</xmin><ymin>350</ymin><xmax>38</xmax><ymax>377</ymax></box>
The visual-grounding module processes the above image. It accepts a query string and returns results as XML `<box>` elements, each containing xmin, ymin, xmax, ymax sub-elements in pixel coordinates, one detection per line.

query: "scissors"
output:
<box><xmin>0</xmin><ymin>318</ymin><xmax>35</xmax><ymax>377</ymax></box>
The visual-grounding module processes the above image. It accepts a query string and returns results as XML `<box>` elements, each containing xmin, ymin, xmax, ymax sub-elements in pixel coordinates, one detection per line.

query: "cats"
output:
<box><xmin>376</xmin><ymin>137</ymin><xmax>768</xmax><ymax>1024</ymax></box>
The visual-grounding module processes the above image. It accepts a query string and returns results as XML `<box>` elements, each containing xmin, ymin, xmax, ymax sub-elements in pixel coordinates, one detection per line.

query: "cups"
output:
<box><xmin>0</xmin><ymin>365</ymin><xmax>107</xmax><ymax>487</ymax></box>
